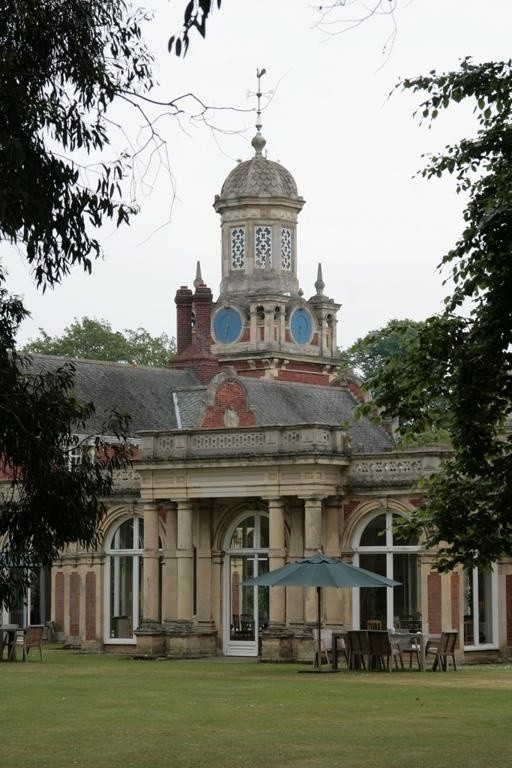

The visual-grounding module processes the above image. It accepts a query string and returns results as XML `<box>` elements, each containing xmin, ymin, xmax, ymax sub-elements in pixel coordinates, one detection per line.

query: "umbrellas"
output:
<box><xmin>240</xmin><ymin>549</ymin><xmax>402</xmax><ymax>672</ymax></box>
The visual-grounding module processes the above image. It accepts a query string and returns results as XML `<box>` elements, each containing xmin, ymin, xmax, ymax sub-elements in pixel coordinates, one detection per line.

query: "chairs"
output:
<box><xmin>229</xmin><ymin>612</ymin><xmax>256</xmax><ymax>641</ymax></box>
<box><xmin>9</xmin><ymin>623</ymin><xmax>49</xmax><ymax>663</ymax></box>
<box><xmin>313</xmin><ymin>610</ymin><xmax>474</xmax><ymax>674</ymax></box>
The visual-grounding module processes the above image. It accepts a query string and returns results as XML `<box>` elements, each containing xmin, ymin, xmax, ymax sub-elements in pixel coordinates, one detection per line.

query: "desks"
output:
<box><xmin>1</xmin><ymin>625</ymin><xmax>31</xmax><ymax>663</ymax></box>
